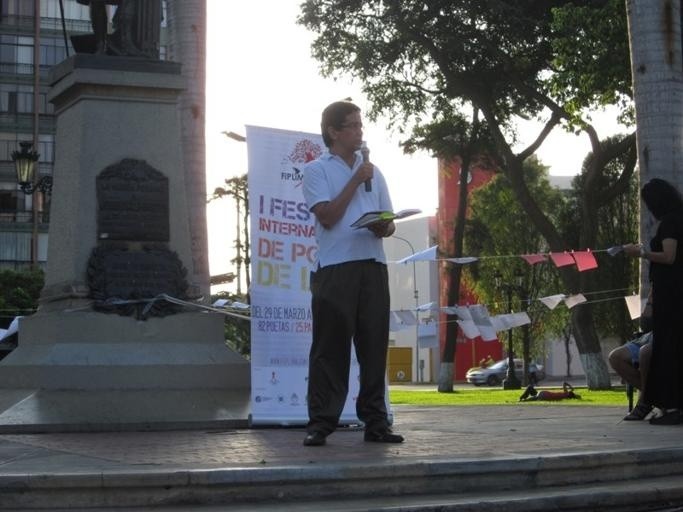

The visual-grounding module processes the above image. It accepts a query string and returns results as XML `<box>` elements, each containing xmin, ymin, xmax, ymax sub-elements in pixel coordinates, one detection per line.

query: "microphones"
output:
<box><xmin>361</xmin><ymin>147</ymin><xmax>371</xmax><ymax>192</ymax></box>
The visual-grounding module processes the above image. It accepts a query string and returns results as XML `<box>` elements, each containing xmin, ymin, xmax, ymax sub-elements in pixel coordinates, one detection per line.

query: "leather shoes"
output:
<box><xmin>624</xmin><ymin>401</ymin><xmax>683</xmax><ymax>425</ymax></box>
<box><xmin>364</xmin><ymin>430</ymin><xmax>403</xmax><ymax>442</ymax></box>
<box><xmin>304</xmin><ymin>433</ymin><xmax>326</xmax><ymax>445</ymax></box>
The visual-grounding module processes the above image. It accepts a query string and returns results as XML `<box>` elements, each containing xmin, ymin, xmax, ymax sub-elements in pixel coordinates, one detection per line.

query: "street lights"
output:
<box><xmin>10</xmin><ymin>141</ymin><xmax>52</xmax><ymax>195</ymax></box>
<box><xmin>396</xmin><ymin>208</ymin><xmax>421</xmax><ymax>220</ymax></box>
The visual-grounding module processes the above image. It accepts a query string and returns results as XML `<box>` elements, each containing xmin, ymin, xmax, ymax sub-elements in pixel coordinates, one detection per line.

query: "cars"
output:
<box><xmin>466</xmin><ymin>357</ymin><xmax>546</xmax><ymax>386</ymax></box>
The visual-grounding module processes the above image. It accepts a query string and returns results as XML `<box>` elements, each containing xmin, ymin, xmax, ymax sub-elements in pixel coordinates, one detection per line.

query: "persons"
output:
<box><xmin>623</xmin><ymin>178</ymin><xmax>683</xmax><ymax>424</ymax></box>
<box><xmin>608</xmin><ymin>279</ymin><xmax>667</xmax><ymax>421</ymax></box>
<box><xmin>520</xmin><ymin>382</ymin><xmax>580</xmax><ymax>401</ymax></box>
<box><xmin>528</xmin><ymin>357</ymin><xmax>540</xmax><ymax>386</ymax></box>
<box><xmin>302</xmin><ymin>102</ymin><xmax>403</xmax><ymax>446</ymax></box>
<box><xmin>78</xmin><ymin>0</ymin><xmax>153</xmax><ymax>57</ymax></box>
<box><xmin>485</xmin><ymin>355</ymin><xmax>495</xmax><ymax>367</ymax></box>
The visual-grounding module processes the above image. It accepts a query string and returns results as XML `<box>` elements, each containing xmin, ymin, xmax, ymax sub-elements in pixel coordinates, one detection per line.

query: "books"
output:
<box><xmin>350</xmin><ymin>208</ymin><xmax>422</xmax><ymax>231</ymax></box>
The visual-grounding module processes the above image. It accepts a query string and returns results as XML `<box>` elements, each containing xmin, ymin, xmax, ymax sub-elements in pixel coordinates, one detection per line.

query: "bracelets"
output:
<box><xmin>640</xmin><ymin>248</ymin><xmax>649</xmax><ymax>258</ymax></box>
<box><xmin>647</xmin><ymin>303</ymin><xmax>653</xmax><ymax>305</ymax></box>
